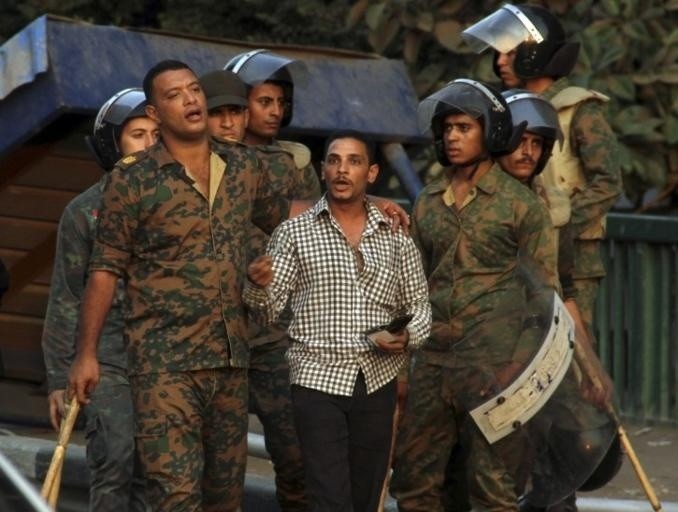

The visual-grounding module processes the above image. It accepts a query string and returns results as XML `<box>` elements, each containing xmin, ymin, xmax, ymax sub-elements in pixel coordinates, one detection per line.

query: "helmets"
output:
<box><xmin>462</xmin><ymin>3</ymin><xmax>566</xmax><ymax>77</ymax></box>
<box><xmin>500</xmin><ymin>88</ymin><xmax>565</xmax><ymax>176</ymax></box>
<box><xmin>223</xmin><ymin>48</ymin><xmax>297</xmax><ymax>129</ymax></box>
<box><xmin>84</xmin><ymin>88</ymin><xmax>151</xmax><ymax>169</ymax></box>
<box><xmin>416</xmin><ymin>78</ymin><xmax>528</xmax><ymax>166</ymax></box>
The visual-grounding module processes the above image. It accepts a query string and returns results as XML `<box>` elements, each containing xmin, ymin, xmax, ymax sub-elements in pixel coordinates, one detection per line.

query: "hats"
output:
<box><xmin>199</xmin><ymin>69</ymin><xmax>249</xmax><ymax>109</ymax></box>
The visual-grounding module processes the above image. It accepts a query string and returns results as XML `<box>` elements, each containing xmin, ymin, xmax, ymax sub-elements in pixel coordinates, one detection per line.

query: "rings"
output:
<box><xmin>391</xmin><ymin>208</ymin><xmax>413</xmax><ymax>220</ymax></box>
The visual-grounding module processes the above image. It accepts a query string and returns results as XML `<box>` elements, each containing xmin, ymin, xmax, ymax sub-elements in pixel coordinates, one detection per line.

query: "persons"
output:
<box><xmin>388</xmin><ymin>78</ymin><xmax>557</xmax><ymax>512</ymax></box>
<box><xmin>497</xmin><ymin>89</ymin><xmax>613</xmax><ymax>512</ymax></box>
<box><xmin>240</xmin><ymin>129</ymin><xmax>433</xmax><ymax>511</ymax></box>
<box><xmin>65</xmin><ymin>60</ymin><xmax>411</xmax><ymax>512</ymax></box>
<box><xmin>462</xmin><ymin>4</ymin><xmax>624</xmax><ymax>349</ymax></box>
<box><xmin>200</xmin><ymin>69</ymin><xmax>308</xmax><ymax>512</ymax></box>
<box><xmin>41</xmin><ymin>88</ymin><xmax>160</xmax><ymax>512</ymax></box>
<box><xmin>221</xmin><ymin>49</ymin><xmax>323</xmax><ymax>207</ymax></box>
